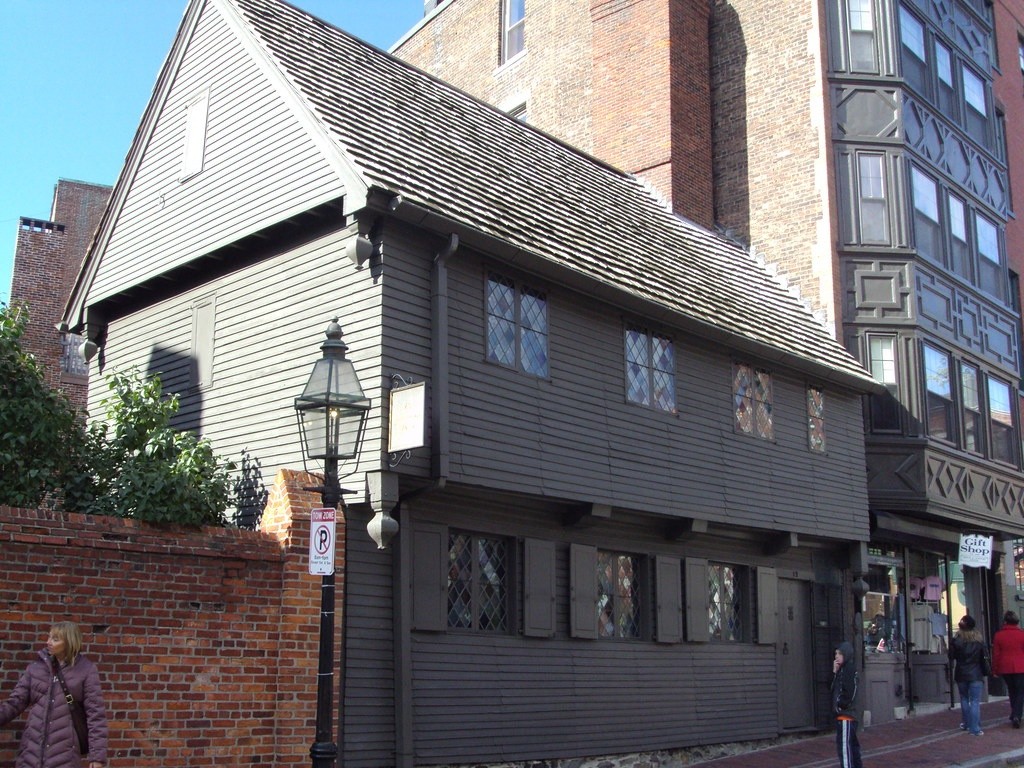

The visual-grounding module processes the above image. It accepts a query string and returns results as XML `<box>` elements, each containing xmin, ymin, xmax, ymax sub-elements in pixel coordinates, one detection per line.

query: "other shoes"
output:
<box><xmin>1013</xmin><ymin>717</ymin><xmax>1020</xmax><ymax>728</ymax></box>
<box><xmin>968</xmin><ymin>730</ymin><xmax>984</xmax><ymax>736</ymax></box>
<box><xmin>960</xmin><ymin>722</ymin><xmax>969</xmax><ymax>730</ymax></box>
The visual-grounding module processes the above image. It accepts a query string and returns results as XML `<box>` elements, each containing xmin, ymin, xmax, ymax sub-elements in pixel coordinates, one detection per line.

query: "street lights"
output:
<box><xmin>293</xmin><ymin>314</ymin><xmax>371</xmax><ymax>768</ymax></box>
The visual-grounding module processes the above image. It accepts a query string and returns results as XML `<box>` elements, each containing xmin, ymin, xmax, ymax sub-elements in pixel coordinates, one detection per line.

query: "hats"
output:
<box><xmin>958</xmin><ymin>615</ymin><xmax>975</xmax><ymax>630</ymax></box>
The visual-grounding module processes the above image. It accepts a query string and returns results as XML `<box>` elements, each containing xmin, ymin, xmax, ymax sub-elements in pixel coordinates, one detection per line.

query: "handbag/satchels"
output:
<box><xmin>69</xmin><ymin>701</ymin><xmax>90</xmax><ymax>755</ymax></box>
<box><xmin>982</xmin><ymin>644</ymin><xmax>992</xmax><ymax>677</ymax></box>
<box><xmin>988</xmin><ymin>674</ymin><xmax>1007</xmax><ymax>697</ymax></box>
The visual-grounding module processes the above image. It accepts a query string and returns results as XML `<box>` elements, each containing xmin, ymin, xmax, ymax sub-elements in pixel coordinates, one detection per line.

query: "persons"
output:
<box><xmin>993</xmin><ymin>610</ymin><xmax>1024</xmax><ymax>729</ymax></box>
<box><xmin>864</xmin><ymin>622</ymin><xmax>879</xmax><ymax>646</ymax></box>
<box><xmin>949</xmin><ymin>615</ymin><xmax>985</xmax><ymax>737</ymax></box>
<box><xmin>832</xmin><ymin>642</ymin><xmax>863</xmax><ymax>768</ymax></box>
<box><xmin>0</xmin><ymin>621</ymin><xmax>109</xmax><ymax>768</ymax></box>
